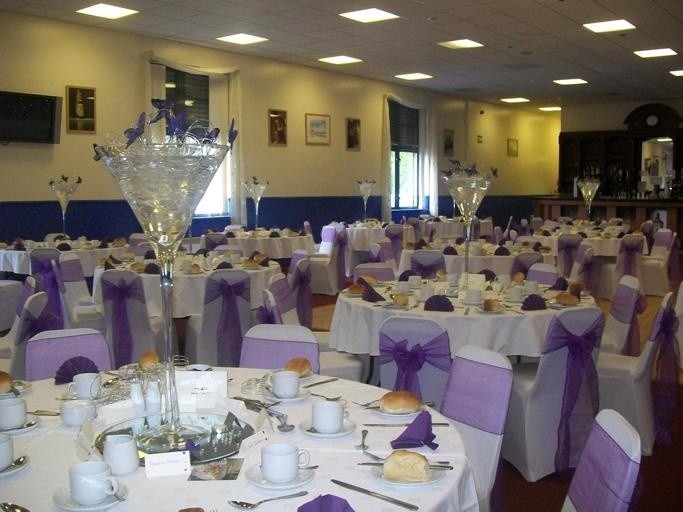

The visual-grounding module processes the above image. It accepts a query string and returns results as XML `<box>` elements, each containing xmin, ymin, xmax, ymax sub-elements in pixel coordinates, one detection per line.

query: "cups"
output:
<box><xmin>524</xmin><ymin>281</ymin><xmax>537</xmax><ymax>296</ymax></box>
<box><xmin>504</xmin><ymin>286</ymin><xmax>522</xmax><ymax>300</ymax></box>
<box><xmin>134</xmin><ymin>255</ymin><xmax>145</xmax><ymax>274</ymax></box>
<box><xmin>580</xmin><ymin>291</ymin><xmax>590</xmax><ymax>304</ymax></box>
<box><xmin>261</xmin><ymin>443</ymin><xmax>310</xmax><ymax>484</ymax></box>
<box><xmin>397</xmin><ymin>281</ymin><xmax>410</xmax><ymax>293</ymax></box>
<box><xmin>0</xmin><ymin>397</ymin><xmax>27</xmax><ymax>431</ymax></box>
<box><xmin>311</xmin><ymin>401</ymin><xmax>343</xmax><ymax>434</ymax></box>
<box><xmin>24</xmin><ymin>240</ymin><xmax>36</xmax><ymax>250</ymax></box>
<box><xmin>182</xmin><ymin>261</ymin><xmax>194</xmax><ymax>272</ymax></box>
<box><xmin>101</xmin><ymin>434</ymin><xmax>140</xmax><ymax>475</ymax></box>
<box><xmin>458</xmin><ymin>289</ymin><xmax>481</xmax><ymax>302</ymax></box>
<box><xmin>72</xmin><ymin>241</ymin><xmax>81</xmax><ymax>250</ymax></box>
<box><xmin>68</xmin><ymin>373</ymin><xmax>102</xmax><ymax>400</ymax></box>
<box><xmin>69</xmin><ymin>461</ymin><xmax>119</xmax><ymax>506</ymax></box>
<box><xmin>229</xmin><ymin>254</ymin><xmax>242</xmax><ymax>265</ymax></box>
<box><xmin>1</xmin><ymin>437</ymin><xmax>15</xmax><ymax>473</ymax></box>
<box><xmin>207</xmin><ymin>252</ymin><xmax>220</xmax><ymax>262</ymax></box>
<box><xmin>91</xmin><ymin>240</ymin><xmax>100</xmax><ymax>248</ymax></box>
<box><xmin>267</xmin><ymin>371</ymin><xmax>300</xmax><ymax>398</ymax></box>
<box><xmin>407</xmin><ymin>275</ymin><xmax>422</xmax><ymax>289</ymax></box>
<box><xmin>59</xmin><ymin>398</ymin><xmax>98</xmax><ymax>429</ymax></box>
<box><xmin>420</xmin><ymin>287</ymin><xmax>434</xmax><ymax>300</ymax></box>
<box><xmin>204</xmin><ymin>258</ymin><xmax>214</xmax><ymax>271</ymax></box>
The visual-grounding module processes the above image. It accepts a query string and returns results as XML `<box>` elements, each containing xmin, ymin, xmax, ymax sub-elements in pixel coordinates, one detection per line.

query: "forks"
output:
<box><xmin>257</xmin><ymin>400</ymin><xmax>282</xmax><ymax>409</ymax></box>
<box><xmin>352</xmin><ymin>400</ymin><xmax>380</xmax><ymax>407</ymax></box>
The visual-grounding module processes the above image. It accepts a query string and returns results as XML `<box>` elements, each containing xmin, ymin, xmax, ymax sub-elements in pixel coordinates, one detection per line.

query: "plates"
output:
<box><xmin>52</xmin><ymin>476</ymin><xmax>129</xmax><ymax>512</ymax></box>
<box><xmin>347</xmin><ymin>293</ymin><xmax>364</xmax><ymax>297</ymax></box>
<box><xmin>118</xmin><ymin>362</ymin><xmax>165</xmax><ymax>377</ymax></box>
<box><xmin>0</xmin><ymin>413</ymin><xmax>42</xmax><ymax>435</ymax></box>
<box><xmin>381</xmin><ymin>461</ymin><xmax>446</xmax><ymax>486</ymax></box>
<box><xmin>375</xmin><ymin>404</ymin><xmax>426</xmax><ymax>417</ymax></box>
<box><xmin>245</xmin><ymin>462</ymin><xmax>315</xmax><ymax>491</ymax></box>
<box><xmin>548</xmin><ymin>298</ymin><xmax>578</xmax><ymax>310</ymax></box>
<box><xmin>298</xmin><ymin>417</ymin><xmax>356</xmax><ymax>437</ymax></box>
<box><xmin>263</xmin><ymin>387</ymin><xmax>311</xmax><ymax>403</ymax></box>
<box><xmin>0</xmin><ymin>450</ymin><xmax>30</xmax><ymax>477</ymax></box>
<box><xmin>506</xmin><ymin>299</ymin><xmax>526</xmax><ymax>303</ymax></box>
<box><xmin>243</xmin><ymin>264</ymin><xmax>263</xmax><ymax>271</ymax></box>
<box><xmin>271</xmin><ymin>368</ymin><xmax>313</xmax><ymax>380</ymax></box>
<box><xmin>0</xmin><ymin>379</ymin><xmax>33</xmax><ymax>398</ymax></box>
<box><xmin>474</xmin><ymin>304</ymin><xmax>504</xmax><ymax>314</ymax></box>
<box><xmin>462</xmin><ymin>299</ymin><xmax>485</xmax><ymax>304</ymax></box>
<box><xmin>416</xmin><ymin>295</ymin><xmax>427</xmax><ymax>302</ymax></box>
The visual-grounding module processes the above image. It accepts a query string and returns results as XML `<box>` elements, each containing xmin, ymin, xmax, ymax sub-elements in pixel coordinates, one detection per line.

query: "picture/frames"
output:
<box><xmin>346</xmin><ymin>117</ymin><xmax>361</xmax><ymax>151</ymax></box>
<box><xmin>67</xmin><ymin>86</ymin><xmax>96</xmax><ymax>133</ymax></box>
<box><xmin>267</xmin><ymin>108</ymin><xmax>288</xmax><ymax>147</ymax></box>
<box><xmin>305</xmin><ymin>114</ymin><xmax>331</xmax><ymax>145</ymax></box>
<box><xmin>444</xmin><ymin>129</ymin><xmax>453</xmax><ymax>157</ymax></box>
<box><xmin>508</xmin><ymin>138</ymin><xmax>518</xmax><ymax>156</ymax></box>
<box><xmin>478</xmin><ymin>136</ymin><xmax>483</xmax><ymax>143</ymax></box>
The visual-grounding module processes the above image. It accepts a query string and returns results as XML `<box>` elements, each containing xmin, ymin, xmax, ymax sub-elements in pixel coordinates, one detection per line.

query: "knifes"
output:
<box><xmin>330</xmin><ymin>478</ymin><xmax>418</xmax><ymax>511</ymax></box>
<box><xmin>365</xmin><ymin>406</ymin><xmax>380</xmax><ymax>409</ymax></box>
<box><xmin>356</xmin><ymin>463</ymin><xmax>453</xmax><ymax>470</ymax></box>
<box><xmin>362</xmin><ymin>423</ymin><xmax>449</xmax><ymax>427</ymax></box>
<box><xmin>302</xmin><ymin>378</ymin><xmax>338</xmax><ymax>388</ymax></box>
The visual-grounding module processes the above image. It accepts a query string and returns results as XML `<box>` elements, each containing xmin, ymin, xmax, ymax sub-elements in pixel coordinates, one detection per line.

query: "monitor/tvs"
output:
<box><xmin>0</xmin><ymin>90</ymin><xmax>62</xmax><ymax>145</ymax></box>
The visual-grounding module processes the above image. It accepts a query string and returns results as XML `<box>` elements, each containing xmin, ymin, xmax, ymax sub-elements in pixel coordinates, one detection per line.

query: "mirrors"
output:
<box><xmin>623</xmin><ymin>103</ymin><xmax>683</xmax><ymax>199</ymax></box>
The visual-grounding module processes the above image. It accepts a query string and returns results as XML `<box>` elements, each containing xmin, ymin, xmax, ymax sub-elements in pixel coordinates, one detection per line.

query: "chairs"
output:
<box><xmin>633</xmin><ymin>223</ymin><xmax>655</xmax><ymax>256</ymax></box>
<box><xmin>559</xmin><ymin>409</ymin><xmax>640</xmax><ymax>512</ymax></box>
<box><xmin>592</xmin><ymin>235</ymin><xmax>644</xmax><ymax>301</ymax></box>
<box><xmin>0</xmin><ymin>276</ymin><xmax>36</xmax><ymax>359</ymax></box>
<box><xmin>489</xmin><ymin>216</ymin><xmax>513</xmax><ymax>242</ymax></box>
<box><xmin>553</xmin><ymin>234</ymin><xmax>583</xmax><ymax>278</ymax></box>
<box><xmin>251</xmin><ymin>259</ymin><xmax>312</xmax><ymax>328</ymax></box>
<box><xmin>29</xmin><ymin>248</ymin><xmax>63</xmax><ymax>329</ymax></box>
<box><xmin>634</xmin><ymin>220</ymin><xmax>652</xmax><ymax>232</ymax></box>
<box><xmin>262</xmin><ymin>288</ymin><xmax>370</xmax><ymax>383</ymax></box>
<box><xmin>411</xmin><ymin>250</ymin><xmax>446</xmax><ymax>280</ymax></box>
<box><xmin>0</xmin><ymin>282</ymin><xmax>35</xmax><ymax>331</ymax></box>
<box><xmin>51</xmin><ymin>259</ymin><xmax>71</xmax><ymax>328</ymax></box>
<box><xmin>311</xmin><ymin>231</ymin><xmax>348</xmax><ymax>296</ymax></box>
<box><xmin>303</xmin><ymin>221</ymin><xmax>311</xmax><ymax>234</ymax></box>
<box><xmin>354</xmin><ymin>263</ymin><xmax>395</xmax><ymax>286</ymax></box>
<box><xmin>269</xmin><ymin>274</ymin><xmax>346</xmax><ymax>353</ymax></box>
<box><xmin>421</xmin><ymin>217</ymin><xmax>432</xmax><ymax>242</ymax></box>
<box><xmin>527</xmin><ymin>263</ymin><xmax>558</xmax><ymax>285</ymax></box>
<box><xmin>288</xmin><ymin>251</ymin><xmax>309</xmax><ymax>275</ymax></box>
<box><xmin>494</xmin><ymin>226</ymin><xmax>514</xmax><ymax>245</ymax></box>
<box><xmin>443</xmin><ymin>346</ymin><xmax>513</xmax><ymax>512</ymax></box>
<box><xmin>101</xmin><ymin>269</ymin><xmax>178</xmax><ymax>371</ymax></box>
<box><xmin>129</xmin><ymin>233</ymin><xmax>150</xmax><ymax>245</ymax></box>
<box><xmin>58</xmin><ymin>251</ymin><xmax>106</xmax><ymax>338</ymax></box>
<box><xmin>186</xmin><ymin>269</ymin><xmax>251</xmax><ymax>367</ymax></box>
<box><xmin>502</xmin><ymin>306</ymin><xmax>605</xmax><ymax>483</ymax></box>
<box><xmin>516</xmin><ymin>236</ymin><xmax>538</xmax><ymax>247</ymax></box>
<box><xmin>215</xmin><ymin>245</ymin><xmax>243</xmax><ymax>257</ymax></box>
<box><xmin>597</xmin><ymin>292</ymin><xmax>673</xmax><ymax>456</ymax></box>
<box><xmin>570</xmin><ymin>249</ymin><xmax>592</xmax><ymax>292</ymax></box>
<box><xmin>642</xmin><ymin>232</ymin><xmax>677</xmax><ymax>297</ymax></box>
<box><xmin>643</xmin><ymin>228</ymin><xmax>671</xmax><ymax>257</ymax></box>
<box><xmin>0</xmin><ymin>292</ymin><xmax>48</xmax><ymax>379</ymax></box>
<box><xmin>385</xmin><ymin>224</ymin><xmax>403</xmax><ymax>252</ymax></box>
<box><xmin>496</xmin><ymin>252</ymin><xmax>544</xmax><ymax>287</ymax></box>
<box><xmin>25</xmin><ymin>328</ymin><xmax>112</xmax><ymax>383</ymax></box>
<box><xmin>307</xmin><ymin>226</ymin><xmax>337</xmax><ymax>258</ymax></box>
<box><xmin>239</xmin><ymin>324</ymin><xmax>320</xmax><ymax>375</ymax></box>
<box><xmin>520</xmin><ymin>219</ymin><xmax>530</xmax><ymax>236</ymax></box>
<box><xmin>533</xmin><ymin>217</ymin><xmax>543</xmax><ymax>230</ymax></box>
<box><xmin>379</xmin><ymin>316</ymin><xmax>451</xmax><ymax>413</ymax></box>
<box><xmin>126</xmin><ymin>242</ymin><xmax>152</xmax><ymax>256</ymax></box>
<box><xmin>206</xmin><ymin>233</ymin><xmax>227</xmax><ymax>253</ymax></box>
<box><xmin>44</xmin><ymin>234</ymin><xmax>69</xmax><ymax>243</ymax></box>
<box><xmin>329</xmin><ymin>222</ymin><xmax>353</xmax><ymax>278</ymax></box>
<box><xmin>375</xmin><ymin>237</ymin><xmax>397</xmax><ymax>272</ymax></box>
<box><xmin>601</xmin><ymin>275</ymin><xmax>646</xmax><ymax>356</ymax></box>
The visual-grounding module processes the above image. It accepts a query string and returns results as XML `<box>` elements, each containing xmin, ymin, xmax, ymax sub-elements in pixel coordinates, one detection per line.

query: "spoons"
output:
<box><xmin>277</xmin><ymin>414</ymin><xmax>295</xmax><ymax>434</ymax></box>
<box><xmin>227</xmin><ymin>491</ymin><xmax>309</xmax><ymax>511</ymax></box>
<box><xmin>355</xmin><ymin>430</ymin><xmax>370</xmax><ymax>452</ymax></box>
<box><xmin>103</xmin><ymin>377</ymin><xmax>119</xmax><ymax>388</ymax></box>
<box><xmin>259</xmin><ymin>465</ymin><xmax>318</xmax><ymax>470</ymax></box>
<box><xmin>0</xmin><ymin>420</ymin><xmax>37</xmax><ymax>432</ymax></box>
<box><xmin>310</xmin><ymin>393</ymin><xmax>342</xmax><ymax>401</ymax></box>
<box><xmin>0</xmin><ymin>503</ymin><xmax>30</xmax><ymax>512</ymax></box>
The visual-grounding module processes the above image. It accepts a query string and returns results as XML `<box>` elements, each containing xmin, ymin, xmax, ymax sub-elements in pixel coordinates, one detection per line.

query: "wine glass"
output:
<box><xmin>576</xmin><ymin>179</ymin><xmax>602</xmax><ymax>227</ymax></box>
<box><xmin>48</xmin><ymin>174</ymin><xmax>83</xmax><ymax>249</ymax></box>
<box><xmin>244</xmin><ymin>178</ymin><xmax>270</xmax><ymax>231</ymax></box>
<box><xmin>357</xmin><ymin>181</ymin><xmax>377</xmax><ymax>224</ymax></box>
<box><xmin>439</xmin><ymin>163</ymin><xmax>500</xmax><ymax>291</ymax></box>
<box><xmin>92</xmin><ymin>143</ymin><xmax>257</xmax><ymax>468</ymax></box>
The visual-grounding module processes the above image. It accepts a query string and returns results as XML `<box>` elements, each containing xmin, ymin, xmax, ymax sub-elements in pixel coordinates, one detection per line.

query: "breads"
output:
<box><xmin>436</xmin><ymin>269</ymin><xmax>445</xmax><ymax>278</ymax></box>
<box><xmin>114</xmin><ymin>237</ymin><xmax>126</xmax><ymax>247</ymax></box>
<box><xmin>0</xmin><ymin>371</ymin><xmax>14</xmax><ymax>394</ymax></box>
<box><xmin>513</xmin><ymin>272</ymin><xmax>525</xmax><ymax>282</ymax></box>
<box><xmin>14</xmin><ymin>237</ymin><xmax>24</xmax><ymax>243</ymax></box>
<box><xmin>120</xmin><ymin>252</ymin><xmax>135</xmax><ymax>260</ymax></box>
<box><xmin>0</xmin><ymin>242</ymin><xmax>7</xmax><ymax>248</ymax></box>
<box><xmin>242</xmin><ymin>259</ymin><xmax>259</xmax><ymax>268</ymax></box>
<box><xmin>224</xmin><ymin>250</ymin><xmax>232</xmax><ymax>258</ymax></box>
<box><xmin>97</xmin><ymin>258</ymin><xmax>112</xmax><ymax>266</ymax></box>
<box><xmin>383</xmin><ymin>450</ymin><xmax>433</xmax><ymax>482</ymax></box>
<box><xmin>484</xmin><ymin>299</ymin><xmax>498</xmax><ymax>311</ymax></box>
<box><xmin>285</xmin><ymin>358</ymin><xmax>312</xmax><ymax>377</ymax></box>
<box><xmin>361</xmin><ymin>275</ymin><xmax>377</xmax><ymax>286</ymax></box>
<box><xmin>556</xmin><ymin>293</ymin><xmax>579</xmax><ymax>306</ymax></box>
<box><xmin>192</xmin><ymin>264</ymin><xmax>200</xmax><ymax>273</ymax></box>
<box><xmin>393</xmin><ymin>293</ymin><xmax>409</xmax><ymax>306</ymax></box>
<box><xmin>348</xmin><ymin>284</ymin><xmax>366</xmax><ymax>294</ymax></box>
<box><xmin>139</xmin><ymin>352</ymin><xmax>159</xmax><ymax>372</ymax></box>
<box><xmin>131</xmin><ymin>262</ymin><xmax>145</xmax><ymax>271</ymax></box>
<box><xmin>254</xmin><ymin>254</ymin><xmax>267</xmax><ymax>263</ymax></box>
<box><xmin>80</xmin><ymin>243</ymin><xmax>93</xmax><ymax>250</ymax></box>
<box><xmin>380</xmin><ymin>391</ymin><xmax>421</xmax><ymax>415</ymax></box>
<box><xmin>569</xmin><ymin>282</ymin><xmax>583</xmax><ymax>294</ymax></box>
<box><xmin>36</xmin><ymin>244</ymin><xmax>42</xmax><ymax>248</ymax></box>
